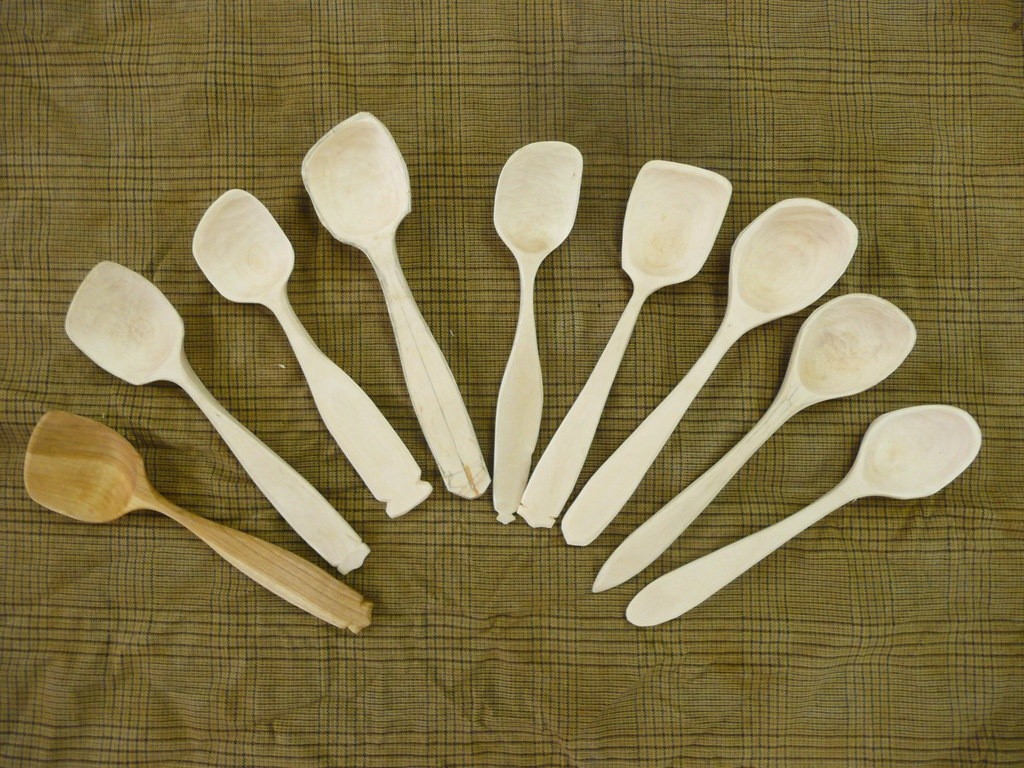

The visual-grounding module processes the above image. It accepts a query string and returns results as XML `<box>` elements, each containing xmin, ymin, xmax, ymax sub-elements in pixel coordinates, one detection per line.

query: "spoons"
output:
<box><xmin>64</xmin><ymin>259</ymin><xmax>370</xmax><ymax>577</ymax></box>
<box><xmin>624</xmin><ymin>404</ymin><xmax>983</xmax><ymax>627</ymax></box>
<box><xmin>191</xmin><ymin>188</ymin><xmax>433</xmax><ymax>521</ymax></box>
<box><xmin>517</xmin><ymin>158</ymin><xmax>735</xmax><ymax>529</ymax></box>
<box><xmin>492</xmin><ymin>140</ymin><xmax>585</xmax><ymax>525</ymax></box>
<box><xmin>590</xmin><ymin>292</ymin><xmax>918</xmax><ymax>594</ymax></box>
<box><xmin>298</xmin><ymin>111</ymin><xmax>491</xmax><ymax>500</ymax></box>
<box><xmin>561</xmin><ymin>198</ymin><xmax>860</xmax><ymax>546</ymax></box>
<box><xmin>22</xmin><ymin>409</ymin><xmax>372</xmax><ymax>637</ymax></box>
